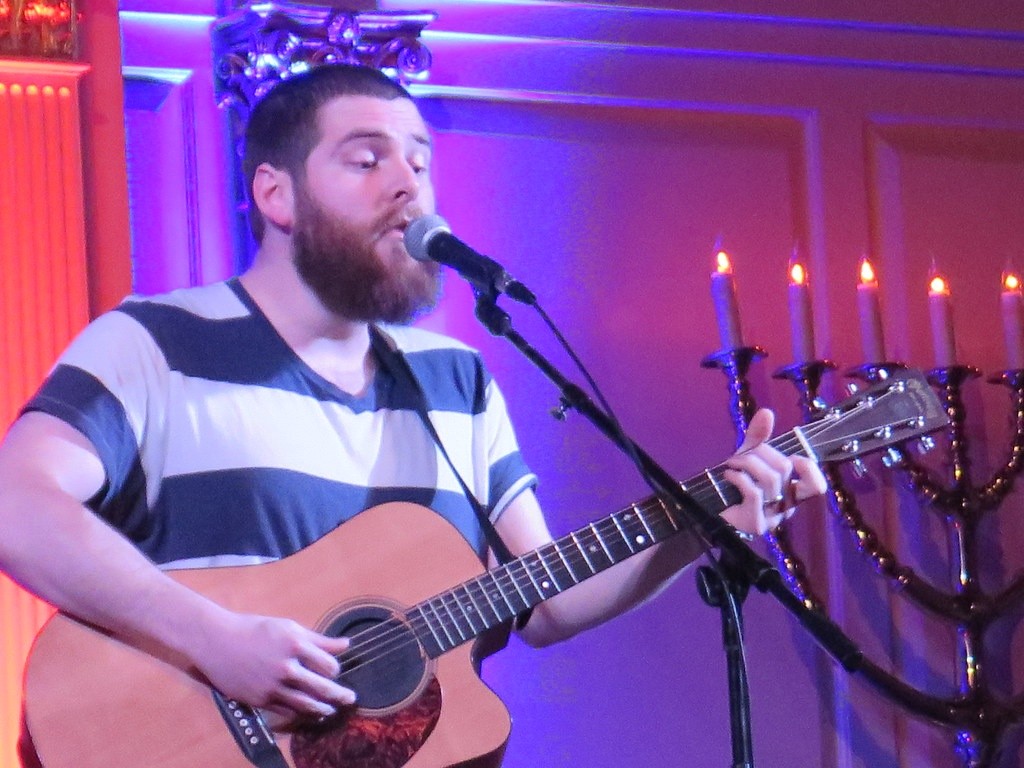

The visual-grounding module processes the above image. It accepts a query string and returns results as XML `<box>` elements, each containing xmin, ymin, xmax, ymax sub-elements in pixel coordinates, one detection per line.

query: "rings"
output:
<box><xmin>764</xmin><ymin>495</ymin><xmax>783</xmax><ymax>506</ymax></box>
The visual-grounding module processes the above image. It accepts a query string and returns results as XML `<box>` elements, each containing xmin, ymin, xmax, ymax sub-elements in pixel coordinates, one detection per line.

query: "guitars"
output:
<box><xmin>15</xmin><ymin>362</ymin><xmax>955</xmax><ymax>768</ymax></box>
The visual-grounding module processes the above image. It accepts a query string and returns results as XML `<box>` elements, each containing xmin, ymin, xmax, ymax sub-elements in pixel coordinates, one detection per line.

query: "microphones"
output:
<box><xmin>401</xmin><ymin>214</ymin><xmax>538</xmax><ymax>305</ymax></box>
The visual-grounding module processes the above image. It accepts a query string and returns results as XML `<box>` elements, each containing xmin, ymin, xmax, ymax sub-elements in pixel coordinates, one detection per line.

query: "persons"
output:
<box><xmin>0</xmin><ymin>61</ymin><xmax>826</xmax><ymax>768</ymax></box>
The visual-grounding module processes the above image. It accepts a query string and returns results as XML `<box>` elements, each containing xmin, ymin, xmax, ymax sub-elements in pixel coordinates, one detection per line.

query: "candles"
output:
<box><xmin>787</xmin><ymin>265</ymin><xmax>817</xmax><ymax>363</ymax></box>
<box><xmin>1001</xmin><ymin>275</ymin><xmax>1023</xmax><ymax>370</ymax></box>
<box><xmin>709</xmin><ymin>251</ymin><xmax>746</xmax><ymax>352</ymax></box>
<box><xmin>926</xmin><ymin>277</ymin><xmax>958</xmax><ymax>366</ymax></box>
<box><xmin>857</xmin><ymin>257</ymin><xmax>886</xmax><ymax>362</ymax></box>
